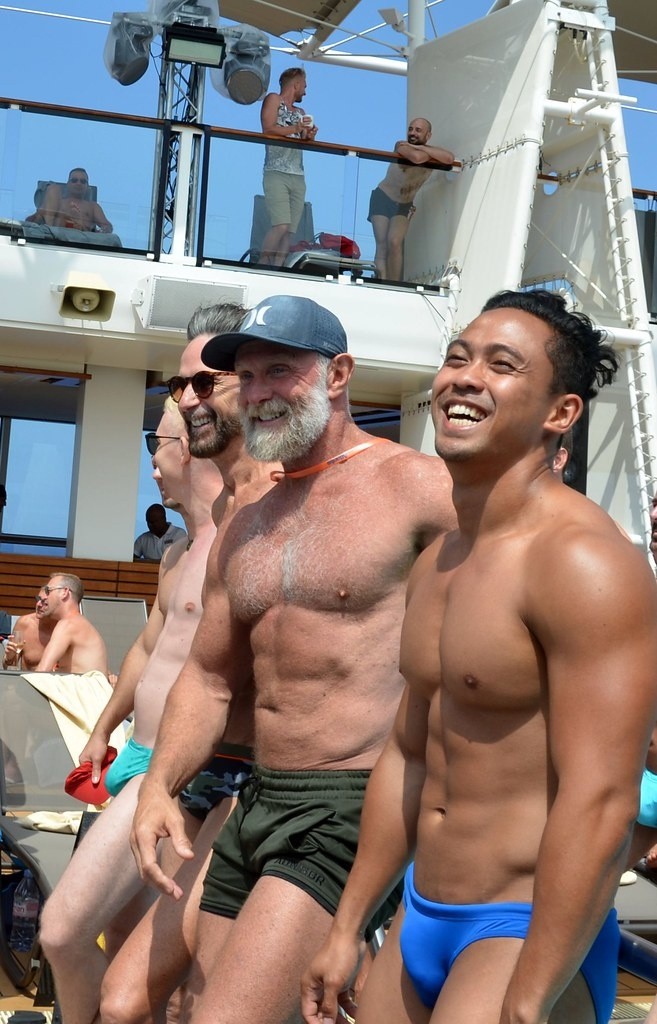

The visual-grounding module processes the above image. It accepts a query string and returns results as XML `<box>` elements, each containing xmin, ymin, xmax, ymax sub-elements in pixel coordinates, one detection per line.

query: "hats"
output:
<box><xmin>201</xmin><ymin>295</ymin><xmax>348</xmax><ymax>372</ymax></box>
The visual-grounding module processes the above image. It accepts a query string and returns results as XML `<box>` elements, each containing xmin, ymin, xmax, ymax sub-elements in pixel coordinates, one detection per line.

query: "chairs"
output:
<box><xmin>248</xmin><ymin>194</ymin><xmax>376</xmax><ymax>278</ymax></box>
<box><xmin>0</xmin><ymin>180</ymin><xmax>122</xmax><ymax>247</ymax></box>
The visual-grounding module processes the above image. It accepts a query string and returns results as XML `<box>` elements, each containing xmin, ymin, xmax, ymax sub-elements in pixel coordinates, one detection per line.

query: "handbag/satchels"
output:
<box><xmin>311</xmin><ymin>231</ymin><xmax>361</xmax><ymax>259</ymax></box>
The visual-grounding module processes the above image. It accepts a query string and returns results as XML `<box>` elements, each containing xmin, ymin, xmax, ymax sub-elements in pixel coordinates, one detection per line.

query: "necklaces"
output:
<box><xmin>186</xmin><ymin>534</ymin><xmax>196</xmax><ymax>550</ymax></box>
<box><xmin>270</xmin><ymin>439</ymin><xmax>391</xmax><ymax>483</ymax></box>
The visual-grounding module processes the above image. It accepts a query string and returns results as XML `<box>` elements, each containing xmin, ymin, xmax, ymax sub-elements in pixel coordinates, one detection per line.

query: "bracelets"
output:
<box><xmin>5</xmin><ymin>659</ymin><xmax>13</xmax><ymax>665</ymax></box>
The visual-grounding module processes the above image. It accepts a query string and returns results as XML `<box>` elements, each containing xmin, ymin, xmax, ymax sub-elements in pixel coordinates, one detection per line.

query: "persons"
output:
<box><xmin>367</xmin><ymin>117</ymin><xmax>455</xmax><ymax>282</ymax></box>
<box><xmin>256</xmin><ymin>67</ymin><xmax>319</xmax><ymax>268</ymax></box>
<box><xmin>298</xmin><ymin>282</ymin><xmax>657</xmax><ymax>1024</ymax></box>
<box><xmin>0</xmin><ymin>572</ymin><xmax>118</xmax><ymax>688</ymax></box>
<box><xmin>25</xmin><ymin>166</ymin><xmax>113</xmax><ymax>233</ymax></box>
<box><xmin>550</xmin><ymin>434</ymin><xmax>657</xmax><ymax>1024</ymax></box>
<box><xmin>130</xmin><ymin>293</ymin><xmax>456</xmax><ymax>1024</ymax></box>
<box><xmin>38</xmin><ymin>303</ymin><xmax>285</xmax><ymax>1024</ymax></box>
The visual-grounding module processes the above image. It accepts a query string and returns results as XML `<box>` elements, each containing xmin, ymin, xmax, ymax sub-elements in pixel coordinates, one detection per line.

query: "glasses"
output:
<box><xmin>33</xmin><ymin>595</ymin><xmax>41</xmax><ymax>602</ymax></box>
<box><xmin>165</xmin><ymin>370</ymin><xmax>238</xmax><ymax>404</ymax></box>
<box><xmin>70</xmin><ymin>178</ymin><xmax>87</xmax><ymax>185</ymax></box>
<box><xmin>144</xmin><ymin>432</ymin><xmax>180</xmax><ymax>455</ymax></box>
<box><xmin>44</xmin><ymin>585</ymin><xmax>72</xmax><ymax>596</ymax></box>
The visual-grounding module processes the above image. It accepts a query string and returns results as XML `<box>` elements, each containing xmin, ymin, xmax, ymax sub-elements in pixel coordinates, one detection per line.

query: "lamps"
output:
<box><xmin>162</xmin><ymin>22</ymin><xmax>226</xmax><ymax>69</ymax></box>
<box><xmin>108</xmin><ymin>22</ymin><xmax>153</xmax><ymax>87</ymax></box>
<box><xmin>224</xmin><ymin>34</ymin><xmax>271</xmax><ymax>105</ymax></box>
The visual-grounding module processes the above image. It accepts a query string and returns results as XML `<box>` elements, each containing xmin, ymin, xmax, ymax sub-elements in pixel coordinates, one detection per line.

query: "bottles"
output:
<box><xmin>9</xmin><ymin>869</ymin><xmax>40</xmax><ymax>953</ymax></box>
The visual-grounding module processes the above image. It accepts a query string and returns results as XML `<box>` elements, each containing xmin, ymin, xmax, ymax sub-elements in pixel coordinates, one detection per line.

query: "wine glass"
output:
<box><xmin>12</xmin><ymin>631</ymin><xmax>23</xmax><ymax>671</ymax></box>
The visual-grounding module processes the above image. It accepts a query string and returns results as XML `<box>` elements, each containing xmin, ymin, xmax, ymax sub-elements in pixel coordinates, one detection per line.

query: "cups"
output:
<box><xmin>301</xmin><ymin>114</ymin><xmax>313</xmax><ymax>128</ymax></box>
